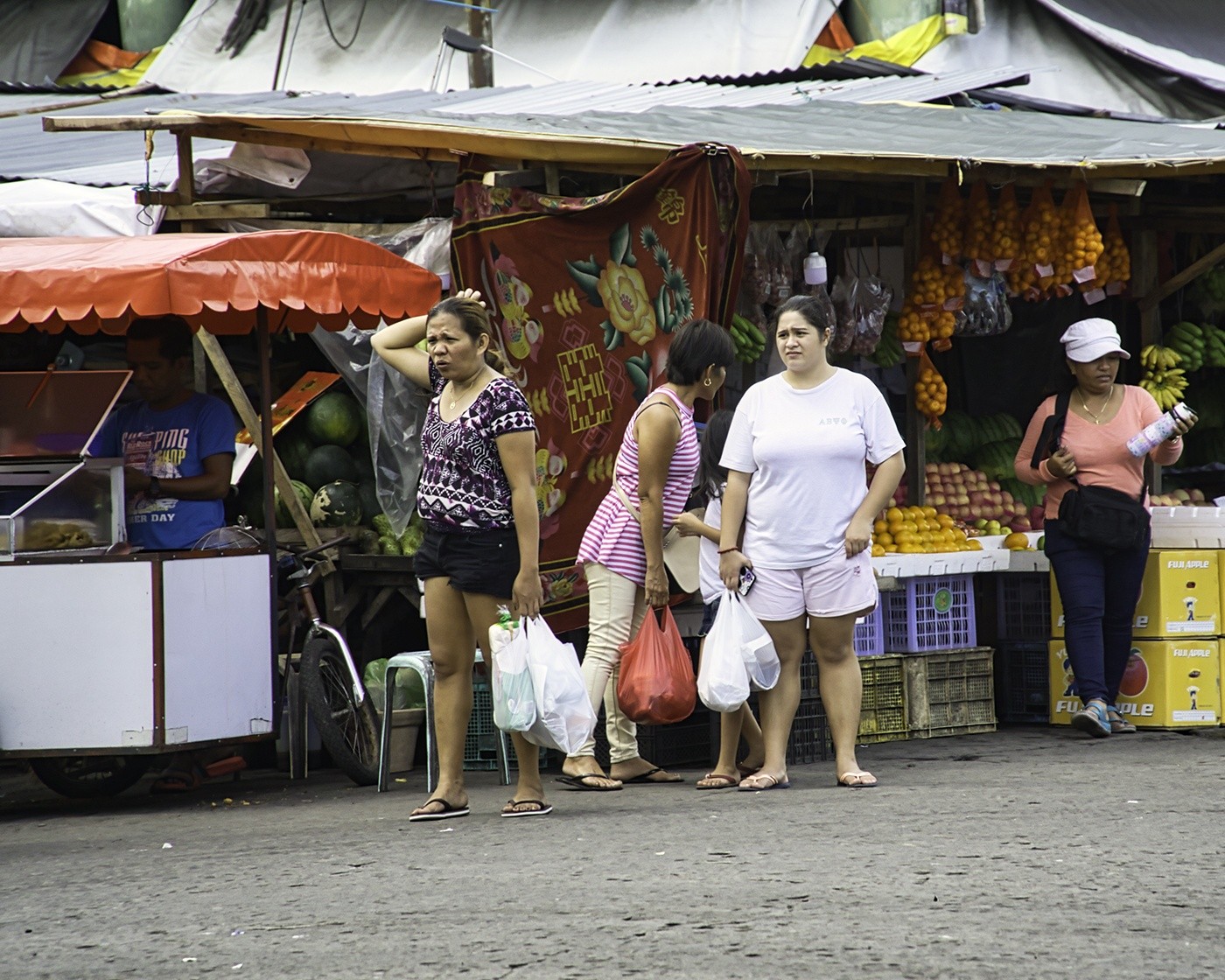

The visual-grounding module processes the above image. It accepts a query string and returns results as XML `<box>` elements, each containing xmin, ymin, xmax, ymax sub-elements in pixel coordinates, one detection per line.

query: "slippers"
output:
<box><xmin>555</xmin><ymin>774</ymin><xmax>623</xmax><ymax>791</ymax></box>
<box><xmin>410</xmin><ymin>799</ymin><xmax>469</xmax><ymax>821</ymax></box>
<box><xmin>148</xmin><ymin>753</ymin><xmax>243</xmax><ymax>791</ymax></box>
<box><xmin>624</xmin><ymin>767</ymin><xmax>685</xmax><ymax>783</ymax></box>
<box><xmin>738</xmin><ymin>775</ymin><xmax>790</xmax><ymax>791</ymax></box>
<box><xmin>697</xmin><ymin>762</ymin><xmax>760</xmax><ymax>790</ymax></box>
<box><xmin>500</xmin><ymin>800</ymin><xmax>553</xmax><ymax>817</ymax></box>
<box><xmin>834</xmin><ymin>772</ymin><xmax>876</xmax><ymax>787</ymax></box>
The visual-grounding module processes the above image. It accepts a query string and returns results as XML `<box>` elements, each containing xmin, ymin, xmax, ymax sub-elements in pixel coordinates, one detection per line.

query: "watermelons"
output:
<box><xmin>925</xmin><ymin>413</ymin><xmax>1050</xmax><ymax>506</ymax></box>
<box><xmin>241</xmin><ymin>388</ymin><xmax>388</xmax><ymax>528</ymax></box>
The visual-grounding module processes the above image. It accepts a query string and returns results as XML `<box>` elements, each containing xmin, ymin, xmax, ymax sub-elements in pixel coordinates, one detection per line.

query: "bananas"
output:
<box><xmin>1137</xmin><ymin>320</ymin><xmax>1225</xmax><ymax>412</ymax></box>
<box><xmin>728</xmin><ymin>312</ymin><xmax>766</xmax><ymax>365</ymax></box>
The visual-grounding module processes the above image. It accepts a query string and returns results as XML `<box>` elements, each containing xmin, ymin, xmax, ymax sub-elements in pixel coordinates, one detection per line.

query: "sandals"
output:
<box><xmin>1107</xmin><ymin>706</ymin><xmax>1137</xmax><ymax>734</ymax></box>
<box><xmin>1070</xmin><ymin>702</ymin><xmax>1111</xmax><ymax>736</ymax></box>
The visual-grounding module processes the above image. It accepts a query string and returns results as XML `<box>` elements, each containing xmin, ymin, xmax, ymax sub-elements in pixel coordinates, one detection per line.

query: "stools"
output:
<box><xmin>377</xmin><ymin>649</ymin><xmax>512</xmax><ymax>792</ymax></box>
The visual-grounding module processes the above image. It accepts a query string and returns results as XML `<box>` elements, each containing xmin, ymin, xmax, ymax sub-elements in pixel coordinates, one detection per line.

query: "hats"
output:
<box><xmin>1058</xmin><ymin>317</ymin><xmax>1131</xmax><ymax>363</ymax></box>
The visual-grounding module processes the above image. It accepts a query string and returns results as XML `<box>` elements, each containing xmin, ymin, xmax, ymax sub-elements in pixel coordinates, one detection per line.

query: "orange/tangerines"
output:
<box><xmin>869</xmin><ymin>505</ymin><xmax>983</xmax><ymax>557</ymax></box>
<box><xmin>890</xmin><ymin>194</ymin><xmax>1131</xmax><ymax>415</ymax></box>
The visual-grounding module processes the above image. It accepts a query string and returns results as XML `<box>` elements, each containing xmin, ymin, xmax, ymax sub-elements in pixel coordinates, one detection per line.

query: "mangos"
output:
<box><xmin>1005</xmin><ymin>532</ymin><xmax>1029</xmax><ymax>548</ymax></box>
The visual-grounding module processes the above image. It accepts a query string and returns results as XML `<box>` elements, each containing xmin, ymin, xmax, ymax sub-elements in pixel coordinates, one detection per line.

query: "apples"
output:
<box><xmin>925</xmin><ymin>462</ymin><xmax>1045</xmax><ymax>536</ymax></box>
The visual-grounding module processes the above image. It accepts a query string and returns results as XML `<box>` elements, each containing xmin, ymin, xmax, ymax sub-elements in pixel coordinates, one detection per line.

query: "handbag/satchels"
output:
<box><xmin>661</xmin><ymin>508</ymin><xmax>705</xmax><ymax>594</ymax></box>
<box><xmin>698</xmin><ymin>588</ymin><xmax>781</xmax><ymax>712</ymax></box>
<box><xmin>617</xmin><ymin>604</ymin><xmax>697</xmax><ymax>724</ymax></box>
<box><xmin>491</xmin><ymin>614</ymin><xmax>599</xmax><ymax>755</ymax></box>
<box><xmin>1058</xmin><ymin>488</ymin><xmax>1145</xmax><ymax>550</ymax></box>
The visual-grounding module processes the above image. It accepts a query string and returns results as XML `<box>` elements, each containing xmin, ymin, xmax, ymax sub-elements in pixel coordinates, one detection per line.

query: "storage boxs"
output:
<box><xmin>462</xmin><ymin>543</ymin><xmax>1011</xmax><ymax>771</ymax></box>
<box><xmin>967</xmin><ymin>506</ymin><xmax>1225</xmax><ymax>730</ymax></box>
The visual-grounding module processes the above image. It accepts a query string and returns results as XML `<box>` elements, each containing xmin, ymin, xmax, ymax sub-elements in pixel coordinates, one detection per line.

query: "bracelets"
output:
<box><xmin>717</xmin><ymin>546</ymin><xmax>739</xmax><ymax>554</ymax></box>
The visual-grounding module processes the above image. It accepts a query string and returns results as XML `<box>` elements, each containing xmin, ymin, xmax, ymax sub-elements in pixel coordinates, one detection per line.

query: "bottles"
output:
<box><xmin>1127</xmin><ymin>402</ymin><xmax>1197</xmax><ymax>458</ymax></box>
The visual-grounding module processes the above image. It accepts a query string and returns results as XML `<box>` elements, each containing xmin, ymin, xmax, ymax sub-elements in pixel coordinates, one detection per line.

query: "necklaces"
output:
<box><xmin>1077</xmin><ymin>385</ymin><xmax>1113</xmax><ymax>424</ymax></box>
<box><xmin>450</xmin><ymin>382</ymin><xmax>474</xmax><ymax>409</ymax></box>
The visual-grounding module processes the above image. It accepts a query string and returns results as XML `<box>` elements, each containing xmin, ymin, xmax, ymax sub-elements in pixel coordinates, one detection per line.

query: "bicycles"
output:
<box><xmin>30</xmin><ymin>531</ymin><xmax>397</xmax><ymax>802</ymax></box>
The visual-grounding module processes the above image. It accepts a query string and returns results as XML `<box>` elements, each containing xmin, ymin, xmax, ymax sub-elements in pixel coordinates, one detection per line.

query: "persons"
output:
<box><xmin>671</xmin><ymin>408</ymin><xmax>766</xmax><ymax>788</ymax></box>
<box><xmin>553</xmin><ymin>318</ymin><xmax>736</xmax><ymax>792</ymax></box>
<box><xmin>714</xmin><ymin>296</ymin><xmax>907</xmax><ymax>792</ymax></box>
<box><xmin>369</xmin><ymin>287</ymin><xmax>553</xmax><ymax>823</ymax></box>
<box><xmin>83</xmin><ymin>313</ymin><xmax>246</xmax><ymax>793</ymax></box>
<box><xmin>1014</xmin><ymin>317</ymin><xmax>1199</xmax><ymax>735</ymax></box>
